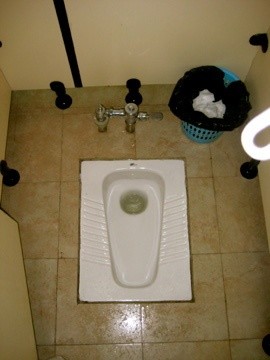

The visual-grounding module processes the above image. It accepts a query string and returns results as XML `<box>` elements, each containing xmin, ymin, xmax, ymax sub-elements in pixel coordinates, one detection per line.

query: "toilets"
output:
<box><xmin>79</xmin><ymin>159</ymin><xmax>192</xmax><ymax>303</ymax></box>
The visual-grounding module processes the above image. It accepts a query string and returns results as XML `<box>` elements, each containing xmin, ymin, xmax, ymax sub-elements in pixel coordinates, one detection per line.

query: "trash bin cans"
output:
<box><xmin>169</xmin><ymin>65</ymin><xmax>252</xmax><ymax>145</ymax></box>
